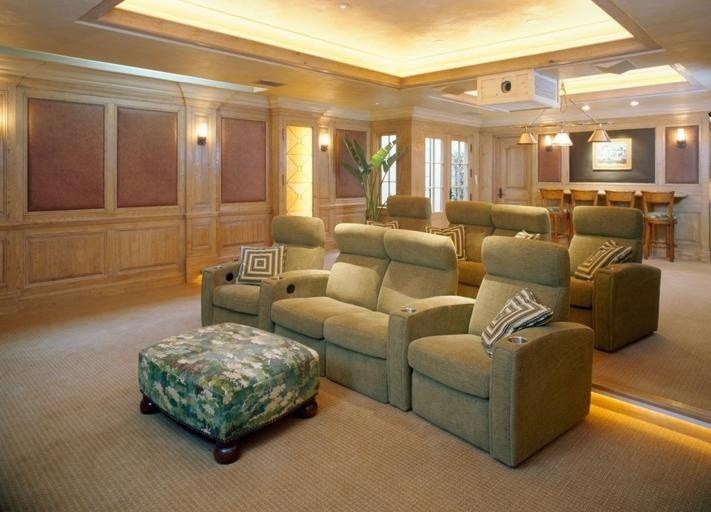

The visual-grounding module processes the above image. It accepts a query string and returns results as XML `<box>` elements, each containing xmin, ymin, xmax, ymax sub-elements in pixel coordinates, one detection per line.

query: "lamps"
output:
<box><xmin>318</xmin><ymin>132</ymin><xmax>334</xmax><ymax>153</ymax></box>
<box><xmin>194</xmin><ymin>120</ymin><xmax>211</xmax><ymax>148</ymax></box>
<box><xmin>516</xmin><ymin>78</ymin><xmax>614</xmax><ymax>148</ymax></box>
<box><xmin>543</xmin><ymin>133</ymin><xmax>551</xmax><ymax>150</ymax></box>
<box><xmin>675</xmin><ymin>126</ymin><xmax>688</xmax><ymax>149</ymax></box>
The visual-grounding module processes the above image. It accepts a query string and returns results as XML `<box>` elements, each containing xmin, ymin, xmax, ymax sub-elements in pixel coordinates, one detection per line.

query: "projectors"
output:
<box><xmin>477</xmin><ymin>68</ymin><xmax>560</xmax><ymax>113</ymax></box>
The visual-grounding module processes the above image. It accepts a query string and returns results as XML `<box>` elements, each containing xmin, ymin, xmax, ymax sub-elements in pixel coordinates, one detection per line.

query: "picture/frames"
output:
<box><xmin>590</xmin><ymin>136</ymin><xmax>636</xmax><ymax>173</ymax></box>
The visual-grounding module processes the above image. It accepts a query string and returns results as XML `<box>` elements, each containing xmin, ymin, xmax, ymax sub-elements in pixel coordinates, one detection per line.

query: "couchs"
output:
<box><xmin>366</xmin><ymin>190</ymin><xmax>661</xmax><ymax>353</ymax></box>
<box><xmin>201</xmin><ymin>215</ymin><xmax>325</xmax><ymax>328</ymax></box>
<box><xmin>136</xmin><ymin>318</ymin><xmax>323</xmax><ymax>466</ymax></box>
<box><xmin>406</xmin><ymin>235</ymin><xmax>593</xmax><ymax>467</ymax></box>
<box><xmin>270</xmin><ymin>223</ymin><xmax>457</xmax><ymax>412</ymax></box>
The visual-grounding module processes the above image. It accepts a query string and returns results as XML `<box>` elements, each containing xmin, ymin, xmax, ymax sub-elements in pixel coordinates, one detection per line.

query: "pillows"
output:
<box><xmin>236</xmin><ymin>244</ymin><xmax>285</xmax><ymax>285</ymax></box>
<box><xmin>482</xmin><ymin>287</ymin><xmax>555</xmax><ymax>358</ymax></box>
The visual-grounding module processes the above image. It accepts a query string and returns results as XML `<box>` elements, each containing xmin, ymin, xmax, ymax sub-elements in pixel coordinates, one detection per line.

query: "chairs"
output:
<box><xmin>537</xmin><ymin>189</ymin><xmax>687</xmax><ymax>262</ymax></box>
